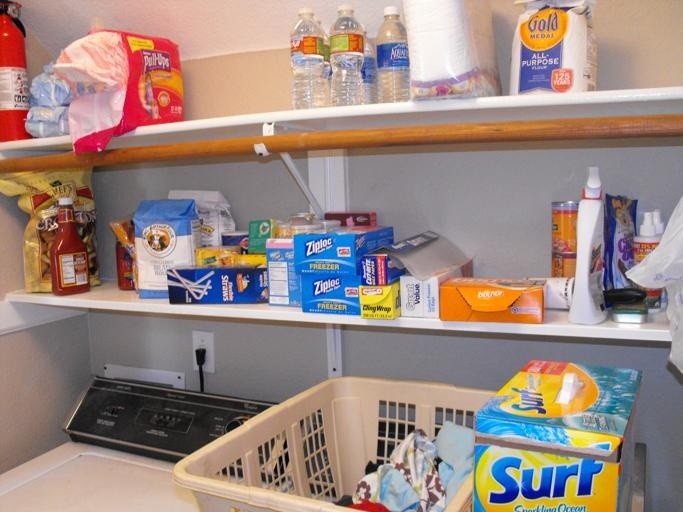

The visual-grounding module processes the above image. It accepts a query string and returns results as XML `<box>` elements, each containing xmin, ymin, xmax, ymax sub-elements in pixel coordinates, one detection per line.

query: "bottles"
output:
<box><xmin>635</xmin><ymin>209</ymin><xmax>667</xmax><ymax>314</ymax></box>
<box><xmin>50</xmin><ymin>197</ymin><xmax>90</xmax><ymax>297</ymax></box>
<box><xmin>290</xmin><ymin>4</ymin><xmax>410</xmax><ymax>110</ymax></box>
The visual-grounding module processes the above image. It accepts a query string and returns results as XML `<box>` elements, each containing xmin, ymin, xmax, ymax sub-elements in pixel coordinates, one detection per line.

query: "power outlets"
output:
<box><xmin>192</xmin><ymin>330</ymin><xmax>215</xmax><ymax>374</ymax></box>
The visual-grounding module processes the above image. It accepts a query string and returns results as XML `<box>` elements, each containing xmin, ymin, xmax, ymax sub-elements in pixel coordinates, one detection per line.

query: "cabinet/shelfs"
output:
<box><xmin>0</xmin><ymin>87</ymin><xmax>683</xmax><ymax>344</ymax></box>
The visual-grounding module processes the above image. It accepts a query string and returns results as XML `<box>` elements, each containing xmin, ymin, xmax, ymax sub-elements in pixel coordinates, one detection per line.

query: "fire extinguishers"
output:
<box><xmin>0</xmin><ymin>0</ymin><xmax>33</xmax><ymax>142</ymax></box>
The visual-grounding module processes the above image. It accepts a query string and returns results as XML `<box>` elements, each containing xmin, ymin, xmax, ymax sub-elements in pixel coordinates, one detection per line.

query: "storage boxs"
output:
<box><xmin>474</xmin><ymin>359</ymin><xmax>643</xmax><ymax>512</ymax></box>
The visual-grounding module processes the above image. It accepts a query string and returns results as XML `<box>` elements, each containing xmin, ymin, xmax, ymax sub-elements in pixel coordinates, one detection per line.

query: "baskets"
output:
<box><xmin>175</xmin><ymin>377</ymin><xmax>499</xmax><ymax>512</ymax></box>
